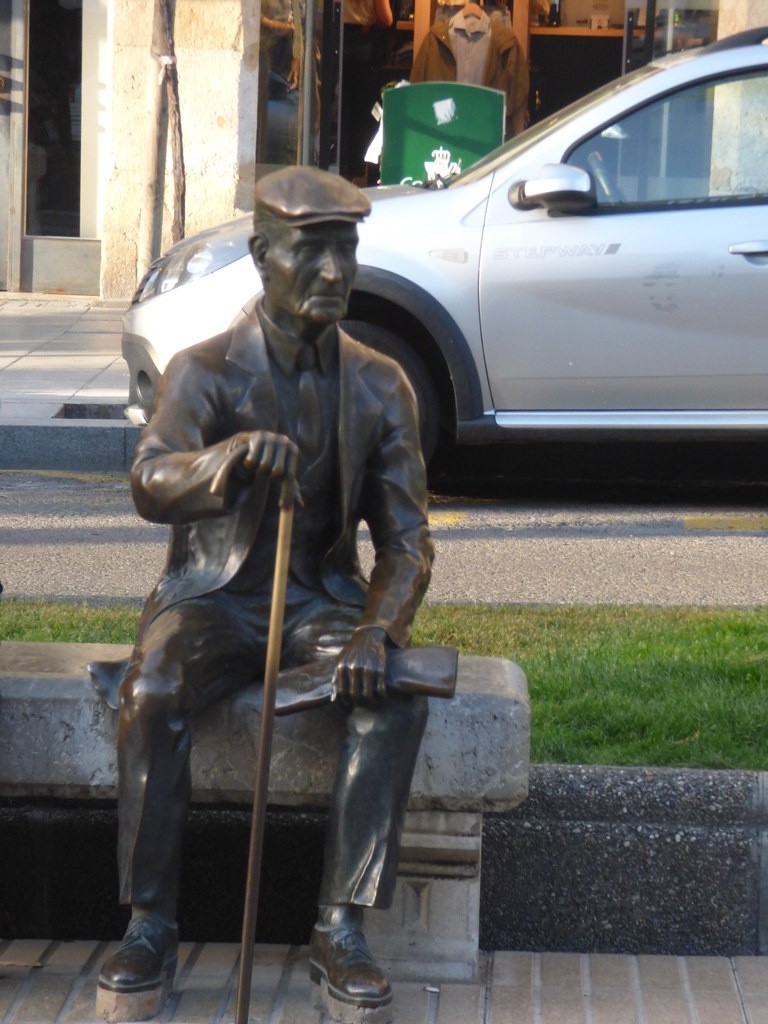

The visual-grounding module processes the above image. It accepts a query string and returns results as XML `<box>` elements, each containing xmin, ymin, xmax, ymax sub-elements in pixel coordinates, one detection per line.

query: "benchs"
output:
<box><xmin>1</xmin><ymin>635</ymin><xmax>529</xmax><ymax>987</ymax></box>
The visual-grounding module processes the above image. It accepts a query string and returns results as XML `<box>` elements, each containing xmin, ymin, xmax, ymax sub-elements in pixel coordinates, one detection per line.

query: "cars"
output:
<box><xmin>122</xmin><ymin>22</ymin><xmax>768</xmax><ymax>485</ymax></box>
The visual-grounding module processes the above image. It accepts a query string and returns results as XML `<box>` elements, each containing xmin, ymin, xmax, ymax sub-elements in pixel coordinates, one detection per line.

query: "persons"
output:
<box><xmin>314</xmin><ymin>0</ymin><xmax>394</xmax><ymax>184</ymax></box>
<box><xmin>96</xmin><ymin>164</ymin><xmax>459</xmax><ymax>1007</ymax></box>
